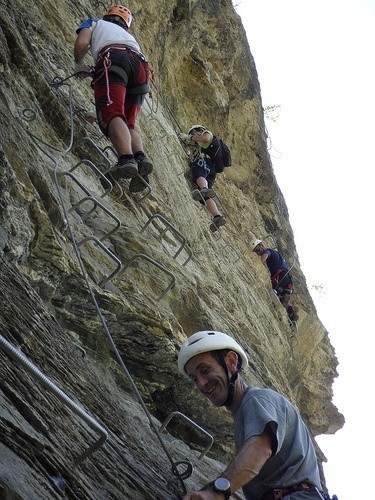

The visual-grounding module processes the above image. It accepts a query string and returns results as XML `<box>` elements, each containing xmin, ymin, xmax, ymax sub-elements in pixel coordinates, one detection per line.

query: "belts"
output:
<box><xmin>261</xmin><ymin>482</ymin><xmax>314</xmax><ymax>500</ymax></box>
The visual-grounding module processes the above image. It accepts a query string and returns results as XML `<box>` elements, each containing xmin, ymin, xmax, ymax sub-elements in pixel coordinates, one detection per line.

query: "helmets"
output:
<box><xmin>188</xmin><ymin>124</ymin><xmax>206</xmax><ymax>135</ymax></box>
<box><xmin>178</xmin><ymin>331</ymin><xmax>248</xmax><ymax>382</ymax></box>
<box><xmin>249</xmin><ymin>238</ymin><xmax>262</xmax><ymax>251</ymax></box>
<box><xmin>103</xmin><ymin>4</ymin><xmax>132</xmax><ymax>31</ymax></box>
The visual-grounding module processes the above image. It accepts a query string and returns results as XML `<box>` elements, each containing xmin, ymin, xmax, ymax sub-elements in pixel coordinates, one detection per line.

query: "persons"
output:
<box><xmin>248</xmin><ymin>238</ymin><xmax>299</xmax><ymax>328</ymax></box>
<box><xmin>178</xmin><ymin>331</ymin><xmax>326</xmax><ymax>500</ymax></box>
<box><xmin>73</xmin><ymin>5</ymin><xmax>154</xmax><ymax>193</ymax></box>
<box><xmin>179</xmin><ymin>125</ymin><xmax>227</xmax><ymax>232</ymax></box>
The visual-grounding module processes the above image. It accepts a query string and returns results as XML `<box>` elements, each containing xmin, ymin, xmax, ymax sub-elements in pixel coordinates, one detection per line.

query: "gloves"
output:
<box><xmin>74</xmin><ymin>61</ymin><xmax>90</xmax><ymax>79</ymax></box>
<box><xmin>180</xmin><ymin>133</ymin><xmax>188</xmax><ymax>142</ymax></box>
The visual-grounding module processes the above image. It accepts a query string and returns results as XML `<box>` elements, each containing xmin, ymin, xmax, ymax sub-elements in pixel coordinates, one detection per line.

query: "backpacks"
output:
<box><xmin>198</xmin><ymin>130</ymin><xmax>232</xmax><ymax>173</ymax></box>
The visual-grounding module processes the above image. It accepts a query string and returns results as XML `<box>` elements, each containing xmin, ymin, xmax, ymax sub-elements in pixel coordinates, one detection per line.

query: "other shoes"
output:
<box><xmin>129</xmin><ymin>153</ymin><xmax>153</xmax><ymax>193</ymax></box>
<box><xmin>193</xmin><ymin>187</ymin><xmax>215</xmax><ymax>200</ymax></box>
<box><xmin>288</xmin><ymin>311</ymin><xmax>299</xmax><ymax>327</ymax></box>
<box><xmin>210</xmin><ymin>216</ymin><xmax>226</xmax><ymax>231</ymax></box>
<box><xmin>99</xmin><ymin>159</ymin><xmax>138</xmax><ymax>189</ymax></box>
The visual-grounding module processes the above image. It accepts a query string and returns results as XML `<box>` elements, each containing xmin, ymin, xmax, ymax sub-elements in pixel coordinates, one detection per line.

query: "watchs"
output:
<box><xmin>199</xmin><ymin>476</ymin><xmax>232</xmax><ymax>500</ymax></box>
